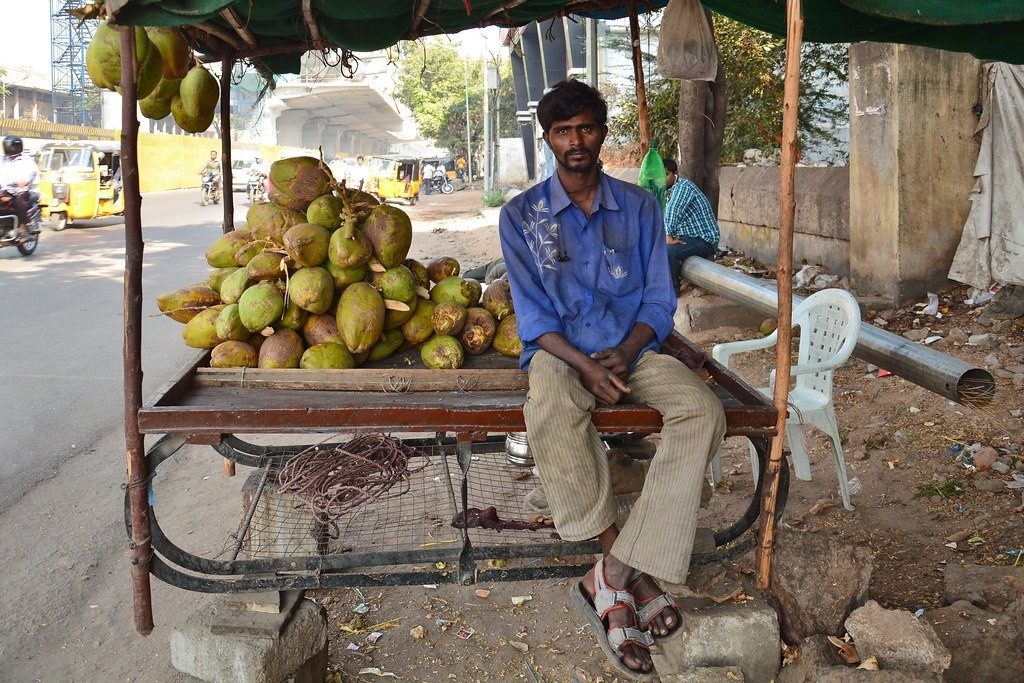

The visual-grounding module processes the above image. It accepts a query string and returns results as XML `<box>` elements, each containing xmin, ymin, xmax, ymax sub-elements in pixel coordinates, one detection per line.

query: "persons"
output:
<box><xmin>499</xmin><ymin>78</ymin><xmax>727</xmax><ymax>681</ymax></box>
<box><xmin>329</xmin><ymin>155</ymin><xmax>368</xmax><ymax>192</ymax></box>
<box><xmin>662</xmin><ymin>158</ymin><xmax>720</xmax><ymax>298</ymax></box>
<box><xmin>456</xmin><ymin>157</ymin><xmax>466</xmax><ymax>179</ymax></box>
<box><xmin>247</xmin><ymin>155</ymin><xmax>269</xmax><ymax>196</ymax></box>
<box><xmin>101</xmin><ymin>155</ymin><xmax>122</xmax><ymax>203</ymax></box>
<box><xmin>423</xmin><ymin>161</ymin><xmax>445</xmax><ymax>195</ymax></box>
<box><xmin>198</xmin><ymin>150</ymin><xmax>223</xmax><ymax>193</ymax></box>
<box><xmin>0</xmin><ymin>135</ymin><xmax>40</xmax><ymax>233</ymax></box>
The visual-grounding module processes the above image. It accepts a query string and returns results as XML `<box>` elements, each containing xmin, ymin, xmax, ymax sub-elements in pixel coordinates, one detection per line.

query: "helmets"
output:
<box><xmin>4</xmin><ymin>136</ymin><xmax>24</xmax><ymax>157</ymax></box>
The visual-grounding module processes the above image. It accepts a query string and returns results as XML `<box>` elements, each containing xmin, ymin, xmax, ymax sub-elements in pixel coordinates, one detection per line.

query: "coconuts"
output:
<box><xmin>156</xmin><ymin>157</ymin><xmax>524</xmax><ymax>371</ymax></box>
<box><xmin>85</xmin><ymin>15</ymin><xmax>219</xmax><ymax>135</ymax></box>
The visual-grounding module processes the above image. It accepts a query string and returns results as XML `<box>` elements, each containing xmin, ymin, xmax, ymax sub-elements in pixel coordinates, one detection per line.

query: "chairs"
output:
<box><xmin>712</xmin><ymin>288</ymin><xmax>861</xmax><ymax>511</ymax></box>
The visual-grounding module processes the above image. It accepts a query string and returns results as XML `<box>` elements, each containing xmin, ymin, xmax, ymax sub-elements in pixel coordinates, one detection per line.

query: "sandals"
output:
<box><xmin>630</xmin><ymin>567</ymin><xmax>683</xmax><ymax>640</ymax></box>
<box><xmin>568</xmin><ymin>556</ymin><xmax>654</xmax><ymax>680</ymax></box>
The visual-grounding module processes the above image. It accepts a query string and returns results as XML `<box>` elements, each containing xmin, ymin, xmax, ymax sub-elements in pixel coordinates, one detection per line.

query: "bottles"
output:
<box><xmin>637</xmin><ymin>139</ymin><xmax>667</xmax><ymax>219</ymax></box>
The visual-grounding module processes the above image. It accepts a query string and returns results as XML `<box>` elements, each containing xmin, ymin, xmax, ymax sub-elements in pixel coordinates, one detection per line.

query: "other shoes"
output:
<box><xmin>14</xmin><ymin>230</ymin><xmax>30</xmax><ymax>242</ymax></box>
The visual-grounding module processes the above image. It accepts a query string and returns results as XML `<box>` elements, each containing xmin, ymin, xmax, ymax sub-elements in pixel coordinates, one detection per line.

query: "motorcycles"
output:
<box><xmin>247</xmin><ymin>171</ymin><xmax>266</xmax><ymax>204</ymax></box>
<box><xmin>421</xmin><ymin>170</ymin><xmax>454</xmax><ymax>195</ymax></box>
<box><xmin>36</xmin><ymin>143</ymin><xmax>125</xmax><ymax>231</ymax></box>
<box><xmin>0</xmin><ymin>183</ymin><xmax>49</xmax><ymax>256</ymax></box>
<box><xmin>199</xmin><ymin>171</ymin><xmax>222</xmax><ymax>206</ymax></box>
<box><xmin>368</xmin><ymin>155</ymin><xmax>423</xmax><ymax>206</ymax></box>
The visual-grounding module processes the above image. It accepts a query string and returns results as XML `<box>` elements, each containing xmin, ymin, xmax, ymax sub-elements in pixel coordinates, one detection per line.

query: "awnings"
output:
<box><xmin>66</xmin><ymin>0</ymin><xmax>1024</xmax><ymax>632</ymax></box>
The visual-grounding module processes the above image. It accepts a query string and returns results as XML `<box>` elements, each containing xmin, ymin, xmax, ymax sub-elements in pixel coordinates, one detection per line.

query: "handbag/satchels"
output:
<box><xmin>656</xmin><ymin>0</ymin><xmax>719</xmax><ymax>83</ymax></box>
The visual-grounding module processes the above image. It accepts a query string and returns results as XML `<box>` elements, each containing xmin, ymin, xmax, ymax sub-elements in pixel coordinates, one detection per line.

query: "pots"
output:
<box><xmin>504</xmin><ymin>431</ymin><xmax>535</xmax><ymax>467</ymax></box>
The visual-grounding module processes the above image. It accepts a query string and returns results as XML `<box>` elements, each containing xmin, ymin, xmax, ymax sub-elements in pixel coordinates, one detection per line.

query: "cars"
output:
<box><xmin>231</xmin><ymin>159</ymin><xmax>256</xmax><ymax>192</ymax></box>
